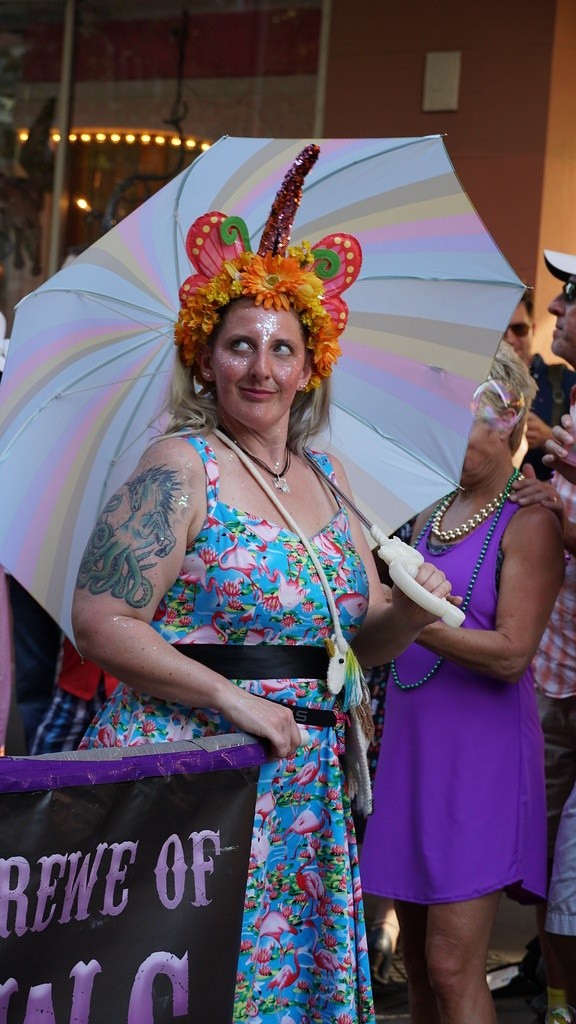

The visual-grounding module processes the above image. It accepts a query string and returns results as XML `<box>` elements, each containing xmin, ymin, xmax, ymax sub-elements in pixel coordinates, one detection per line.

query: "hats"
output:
<box><xmin>544</xmin><ymin>250</ymin><xmax>576</xmax><ymax>282</ymax></box>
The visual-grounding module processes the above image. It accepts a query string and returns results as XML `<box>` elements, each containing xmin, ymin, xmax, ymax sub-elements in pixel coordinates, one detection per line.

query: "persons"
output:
<box><xmin>0</xmin><ymin>568</ymin><xmax>121</xmax><ymax>753</ymax></box>
<box><xmin>504</xmin><ymin>249</ymin><xmax>575</xmax><ymax>1024</ymax></box>
<box><xmin>359</xmin><ymin>513</ymin><xmax>417</xmax><ymax>1001</ymax></box>
<box><xmin>71</xmin><ymin>145</ymin><xmax>462</xmax><ymax>1024</ymax></box>
<box><xmin>358</xmin><ymin>340</ymin><xmax>562</xmax><ymax>1024</ymax></box>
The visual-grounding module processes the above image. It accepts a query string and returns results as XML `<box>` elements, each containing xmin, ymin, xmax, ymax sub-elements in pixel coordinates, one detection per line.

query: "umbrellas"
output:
<box><xmin>0</xmin><ymin>133</ymin><xmax>536</xmax><ymax>660</ymax></box>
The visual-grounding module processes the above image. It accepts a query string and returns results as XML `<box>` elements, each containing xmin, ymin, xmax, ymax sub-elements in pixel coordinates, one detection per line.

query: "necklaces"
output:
<box><xmin>392</xmin><ymin>466</ymin><xmax>520</xmax><ymax>689</ymax></box>
<box><xmin>221</xmin><ymin>426</ymin><xmax>291</xmax><ymax>493</ymax></box>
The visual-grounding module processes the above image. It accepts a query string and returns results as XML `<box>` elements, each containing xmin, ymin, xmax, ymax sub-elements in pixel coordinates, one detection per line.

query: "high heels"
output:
<box><xmin>366</xmin><ymin>926</ymin><xmax>392</xmax><ymax>982</ymax></box>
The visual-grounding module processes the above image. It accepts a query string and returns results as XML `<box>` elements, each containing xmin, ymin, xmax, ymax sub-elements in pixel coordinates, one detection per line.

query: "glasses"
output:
<box><xmin>563</xmin><ymin>282</ymin><xmax>576</xmax><ymax>301</ymax></box>
<box><xmin>505</xmin><ymin>323</ymin><xmax>534</xmax><ymax>337</ymax></box>
